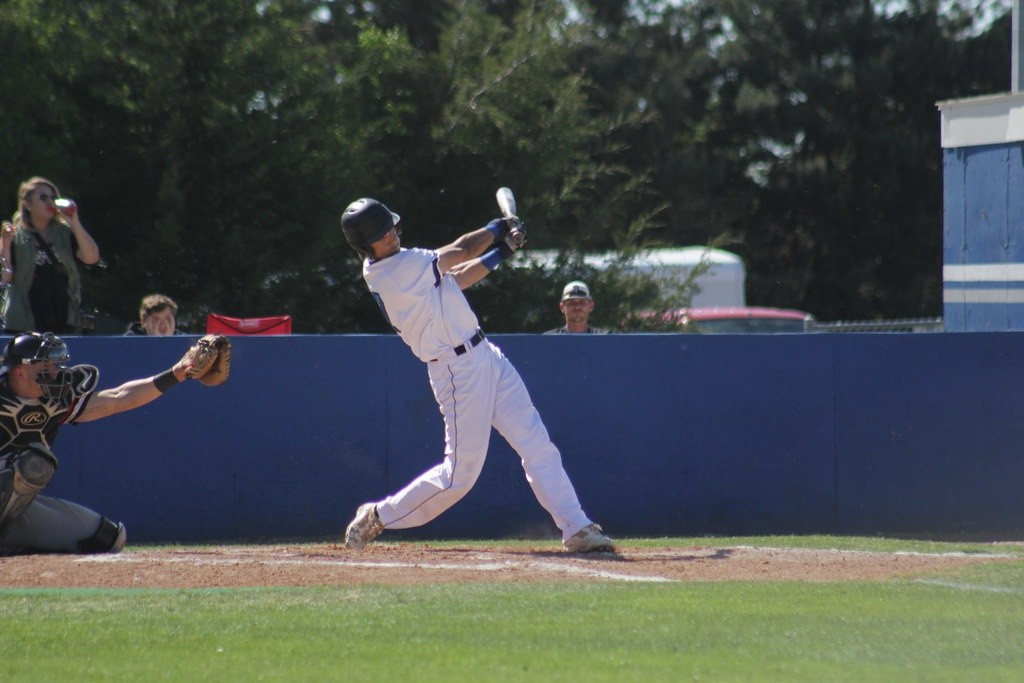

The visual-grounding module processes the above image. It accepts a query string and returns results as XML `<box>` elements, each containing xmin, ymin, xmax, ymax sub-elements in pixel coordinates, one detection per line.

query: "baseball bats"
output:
<box><xmin>495</xmin><ymin>185</ymin><xmax>524</xmax><ymax>248</ymax></box>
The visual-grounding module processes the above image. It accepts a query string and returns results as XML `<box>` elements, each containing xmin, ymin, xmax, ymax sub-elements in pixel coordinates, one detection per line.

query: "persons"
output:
<box><xmin>339</xmin><ymin>197</ymin><xmax>616</xmax><ymax>552</ymax></box>
<box><xmin>124</xmin><ymin>294</ymin><xmax>189</xmax><ymax>337</ymax></box>
<box><xmin>0</xmin><ymin>176</ymin><xmax>99</xmax><ymax>336</ymax></box>
<box><xmin>540</xmin><ymin>280</ymin><xmax>611</xmax><ymax>336</ymax></box>
<box><xmin>0</xmin><ymin>331</ymin><xmax>229</xmax><ymax>558</ymax></box>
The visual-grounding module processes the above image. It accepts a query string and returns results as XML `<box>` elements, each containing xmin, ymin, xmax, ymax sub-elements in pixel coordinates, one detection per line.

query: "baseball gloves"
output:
<box><xmin>180</xmin><ymin>332</ymin><xmax>231</xmax><ymax>387</ymax></box>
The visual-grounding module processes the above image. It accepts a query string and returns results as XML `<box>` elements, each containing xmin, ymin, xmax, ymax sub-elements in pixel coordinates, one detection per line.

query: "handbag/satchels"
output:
<box><xmin>74</xmin><ymin>308</ymin><xmax>97</xmax><ymax>332</ymax></box>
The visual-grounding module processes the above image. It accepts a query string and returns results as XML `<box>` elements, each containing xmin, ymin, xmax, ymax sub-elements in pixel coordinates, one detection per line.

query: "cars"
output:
<box><xmin>644</xmin><ymin>308</ymin><xmax>818</xmax><ymax>334</ymax></box>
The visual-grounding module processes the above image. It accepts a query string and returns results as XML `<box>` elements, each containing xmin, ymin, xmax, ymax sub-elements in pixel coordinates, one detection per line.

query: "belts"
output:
<box><xmin>454</xmin><ymin>330</ymin><xmax>485</xmax><ymax>355</ymax></box>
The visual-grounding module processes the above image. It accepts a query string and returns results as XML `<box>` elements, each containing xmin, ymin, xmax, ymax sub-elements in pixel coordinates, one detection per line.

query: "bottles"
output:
<box><xmin>47</xmin><ymin>198</ymin><xmax>77</xmax><ymax>215</ymax></box>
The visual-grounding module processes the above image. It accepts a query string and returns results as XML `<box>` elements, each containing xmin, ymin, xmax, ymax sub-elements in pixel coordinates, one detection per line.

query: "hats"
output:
<box><xmin>561</xmin><ymin>280</ymin><xmax>592</xmax><ymax>301</ymax></box>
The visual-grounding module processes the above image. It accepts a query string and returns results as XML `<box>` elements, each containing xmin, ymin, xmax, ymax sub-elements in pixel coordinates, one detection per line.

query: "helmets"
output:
<box><xmin>0</xmin><ymin>331</ymin><xmax>55</xmax><ymax>381</ymax></box>
<box><xmin>341</xmin><ymin>198</ymin><xmax>402</xmax><ymax>258</ymax></box>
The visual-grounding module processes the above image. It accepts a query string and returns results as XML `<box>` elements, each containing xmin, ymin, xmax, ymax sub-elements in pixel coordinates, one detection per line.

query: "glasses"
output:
<box><xmin>28</xmin><ymin>194</ymin><xmax>59</xmax><ymax>202</ymax></box>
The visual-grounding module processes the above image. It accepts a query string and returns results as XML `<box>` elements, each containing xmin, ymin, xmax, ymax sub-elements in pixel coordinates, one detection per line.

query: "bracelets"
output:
<box><xmin>486</xmin><ymin>224</ymin><xmax>498</xmax><ymax>242</ymax></box>
<box><xmin>480</xmin><ymin>248</ymin><xmax>502</xmax><ymax>272</ymax></box>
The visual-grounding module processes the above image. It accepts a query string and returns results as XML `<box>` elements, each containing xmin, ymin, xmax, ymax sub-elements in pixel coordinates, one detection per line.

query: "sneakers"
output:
<box><xmin>569</xmin><ymin>526</ymin><xmax>617</xmax><ymax>554</ymax></box>
<box><xmin>345</xmin><ymin>500</ymin><xmax>383</xmax><ymax>544</ymax></box>
